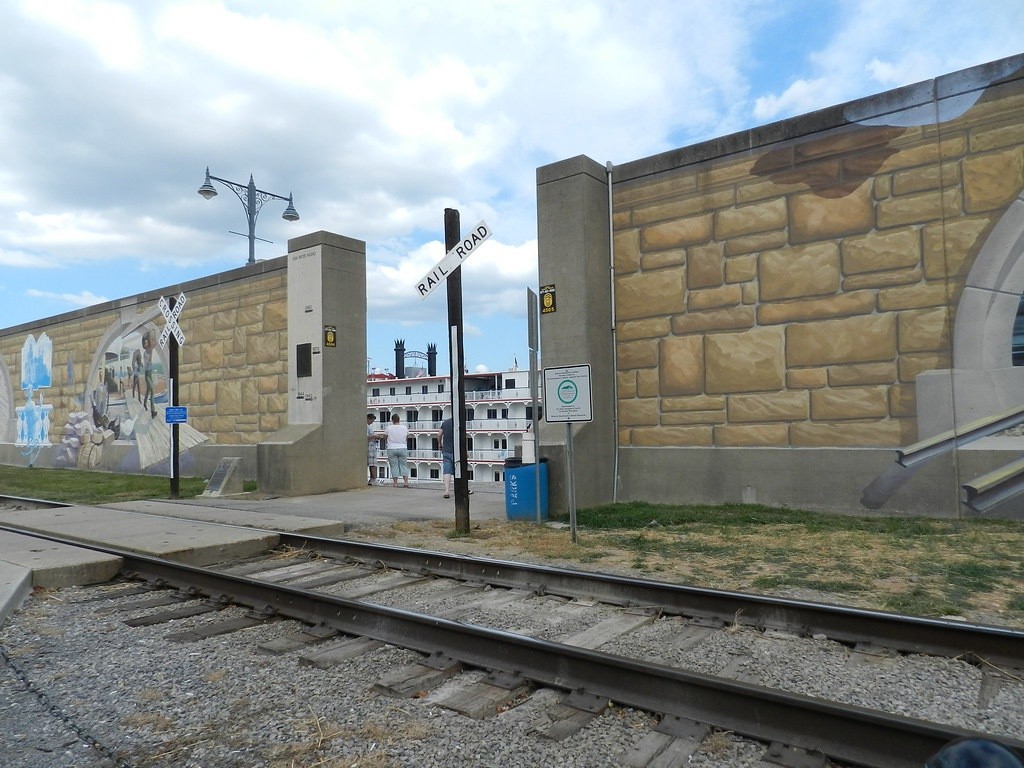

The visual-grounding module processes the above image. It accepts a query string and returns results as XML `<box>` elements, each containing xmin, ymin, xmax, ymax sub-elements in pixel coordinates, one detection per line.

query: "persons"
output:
<box><xmin>437</xmin><ymin>418</ymin><xmax>474</xmax><ymax>498</ymax></box>
<box><xmin>383</xmin><ymin>413</ymin><xmax>409</xmax><ymax>487</ymax></box>
<box><xmin>367</xmin><ymin>413</ymin><xmax>382</xmax><ymax>486</ymax></box>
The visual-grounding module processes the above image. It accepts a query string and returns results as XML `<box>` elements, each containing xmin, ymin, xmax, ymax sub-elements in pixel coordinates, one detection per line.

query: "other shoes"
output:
<box><xmin>468</xmin><ymin>490</ymin><xmax>474</xmax><ymax>494</ymax></box>
<box><xmin>368</xmin><ymin>478</ymin><xmax>384</xmax><ymax>486</ymax></box>
<box><xmin>393</xmin><ymin>484</ymin><xmax>398</xmax><ymax>487</ymax></box>
<box><xmin>444</xmin><ymin>495</ymin><xmax>450</xmax><ymax>498</ymax></box>
<box><xmin>403</xmin><ymin>484</ymin><xmax>409</xmax><ymax>488</ymax></box>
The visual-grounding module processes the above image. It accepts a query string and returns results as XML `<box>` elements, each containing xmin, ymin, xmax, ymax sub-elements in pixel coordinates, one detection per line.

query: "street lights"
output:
<box><xmin>197</xmin><ymin>164</ymin><xmax>300</xmax><ymax>266</ymax></box>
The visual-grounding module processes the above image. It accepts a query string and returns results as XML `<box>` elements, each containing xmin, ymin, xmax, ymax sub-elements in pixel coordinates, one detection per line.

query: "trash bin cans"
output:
<box><xmin>504</xmin><ymin>456</ymin><xmax>549</xmax><ymax>521</ymax></box>
